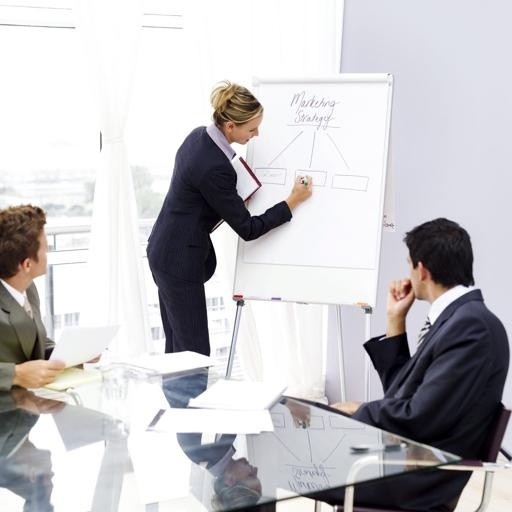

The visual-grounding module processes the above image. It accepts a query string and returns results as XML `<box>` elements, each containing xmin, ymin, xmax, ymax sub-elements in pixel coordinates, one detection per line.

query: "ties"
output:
<box><xmin>416</xmin><ymin>317</ymin><xmax>430</xmax><ymax>347</ymax></box>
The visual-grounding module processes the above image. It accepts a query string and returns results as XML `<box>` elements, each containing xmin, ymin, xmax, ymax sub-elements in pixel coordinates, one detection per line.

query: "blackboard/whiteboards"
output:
<box><xmin>232</xmin><ymin>73</ymin><xmax>395</xmax><ymax>308</ymax></box>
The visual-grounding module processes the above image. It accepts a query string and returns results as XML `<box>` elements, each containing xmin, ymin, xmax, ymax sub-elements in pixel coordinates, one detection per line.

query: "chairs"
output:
<box><xmin>315</xmin><ymin>403</ymin><xmax>512</xmax><ymax>512</ymax></box>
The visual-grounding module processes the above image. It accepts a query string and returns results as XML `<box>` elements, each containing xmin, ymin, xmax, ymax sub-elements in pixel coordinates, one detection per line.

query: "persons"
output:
<box><xmin>145</xmin><ymin>79</ymin><xmax>314</xmax><ymax>352</ymax></box>
<box><xmin>0</xmin><ymin>204</ymin><xmax>100</xmax><ymax>389</ymax></box>
<box><xmin>0</xmin><ymin>389</ymin><xmax>66</xmax><ymax>512</ymax></box>
<box><xmin>157</xmin><ymin>372</ymin><xmax>311</xmax><ymax>512</ymax></box>
<box><xmin>233</xmin><ymin>217</ymin><xmax>510</xmax><ymax>512</ymax></box>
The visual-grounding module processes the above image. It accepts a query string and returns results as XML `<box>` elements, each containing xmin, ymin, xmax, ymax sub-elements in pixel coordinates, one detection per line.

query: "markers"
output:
<box><xmin>302</xmin><ymin>174</ymin><xmax>309</xmax><ymax>186</ymax></box>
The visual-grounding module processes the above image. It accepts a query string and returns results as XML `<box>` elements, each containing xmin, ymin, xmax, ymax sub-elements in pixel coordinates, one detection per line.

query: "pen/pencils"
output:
<box><xmin>350</xmin><ymin>442</ymin><xmax>406</xmax><ymax>453</ymax></box>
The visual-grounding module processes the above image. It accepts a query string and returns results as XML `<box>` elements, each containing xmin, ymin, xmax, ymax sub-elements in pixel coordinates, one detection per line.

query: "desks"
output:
<box><xmin>0</xmin><ymin>363</ymin><xmax>460</xmax><ymax>512</ymax></box>
